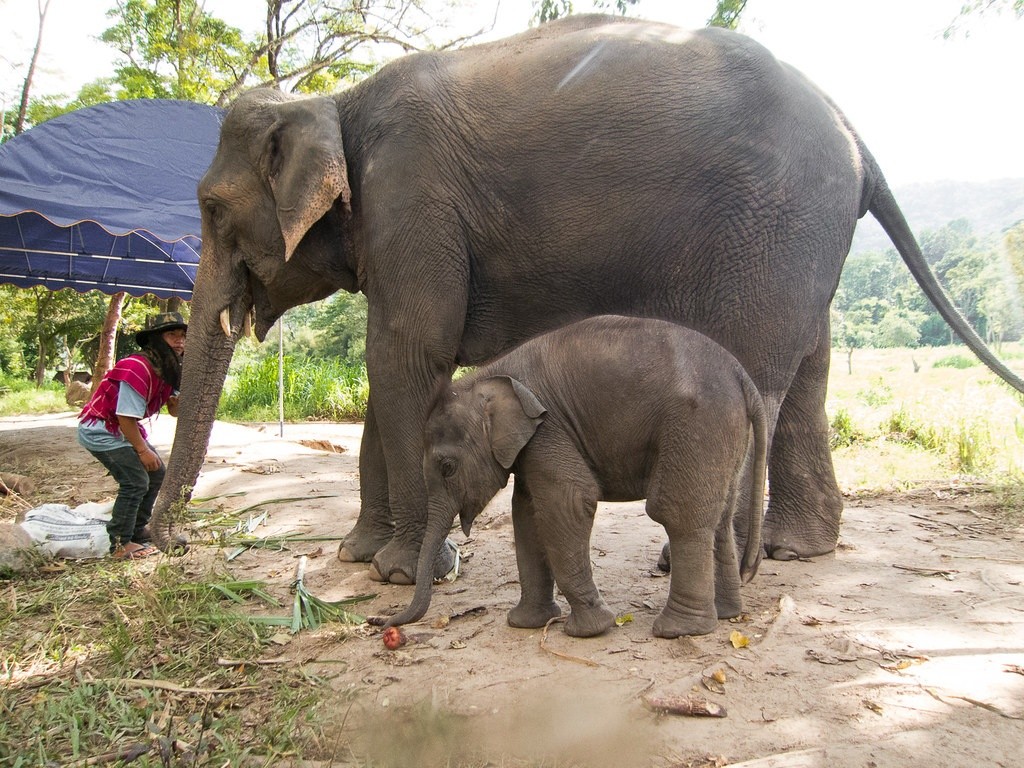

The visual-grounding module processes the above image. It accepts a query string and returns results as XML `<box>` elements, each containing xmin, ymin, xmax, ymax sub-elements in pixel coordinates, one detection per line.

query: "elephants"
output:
<box><xmin>365</xmin><ymin>314</ymin><xmax>768</xmax><ymax>639</ymax></box>
<box><xmin>149</xmin><ymin>13</ymin><xmax>1024</xmax><ymax>585</ymax></box>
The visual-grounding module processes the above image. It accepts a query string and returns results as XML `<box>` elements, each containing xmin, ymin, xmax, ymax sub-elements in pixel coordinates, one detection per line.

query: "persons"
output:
<box><xmin>76</xmin><ymin>310</ymin><xmax>189</xmax><ymax>559</ymax></box>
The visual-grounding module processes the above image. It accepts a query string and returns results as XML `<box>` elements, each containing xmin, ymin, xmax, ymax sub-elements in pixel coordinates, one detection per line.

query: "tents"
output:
<box><xmin>0</xmin><ymin>99</ymin><xmax>289</xmax><ymax>444</ymax></box>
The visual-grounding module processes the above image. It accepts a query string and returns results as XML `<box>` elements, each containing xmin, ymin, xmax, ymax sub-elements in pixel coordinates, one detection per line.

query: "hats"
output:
<box><xmin>136</xmin><ymin>312</ymin><xmax>188</xmax><ymax>349</ymax></box>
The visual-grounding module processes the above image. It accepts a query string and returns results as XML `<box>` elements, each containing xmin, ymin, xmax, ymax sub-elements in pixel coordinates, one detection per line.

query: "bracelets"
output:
<box><xmin>138</xmin><ymin>448</ymin><xmax>151</xmax><ymax>455</ymax></box>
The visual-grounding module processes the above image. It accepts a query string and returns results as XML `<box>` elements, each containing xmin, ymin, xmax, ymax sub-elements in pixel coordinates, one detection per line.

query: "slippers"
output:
<box><xmin>142</xmin><ymin>544</ymin><xmax>160</xmax><ymax>555</ymax></box>
<box><xmin>108</xmin><ymin>544</ymin><xmax>148</xmax><ymax>559</ymax></box>
